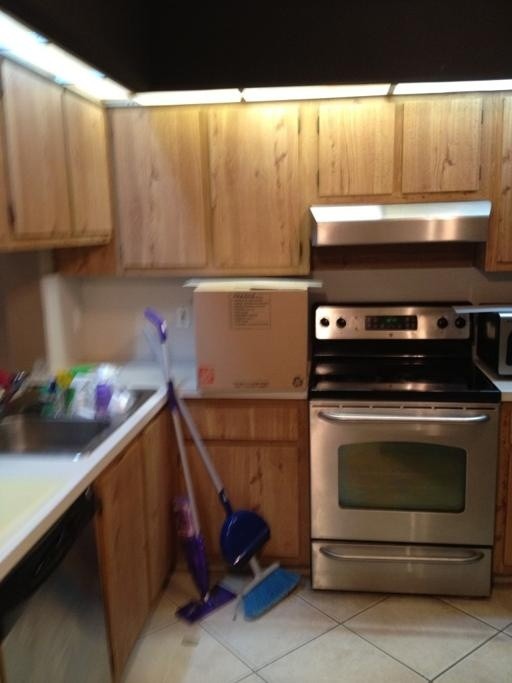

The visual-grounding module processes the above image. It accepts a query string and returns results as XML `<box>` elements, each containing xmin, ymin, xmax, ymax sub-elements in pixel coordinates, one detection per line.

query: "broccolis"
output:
<box><xmin>0</xmin><ymin>486</ymin><xmax>112</xmax><ymax>682</ymax></box>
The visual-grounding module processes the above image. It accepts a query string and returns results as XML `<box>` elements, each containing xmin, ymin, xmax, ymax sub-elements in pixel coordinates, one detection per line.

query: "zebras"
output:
<box><xmin>310</xmin><ymin>398</ymin><xmax>493</xmax><ymax>598</ymax></box>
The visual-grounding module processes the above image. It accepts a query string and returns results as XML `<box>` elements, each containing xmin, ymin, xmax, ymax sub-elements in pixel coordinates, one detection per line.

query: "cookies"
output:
<box><xmin>479</xmin><ymin>312</ymin><xmax>512</xmax><ymax>377</ymax></box>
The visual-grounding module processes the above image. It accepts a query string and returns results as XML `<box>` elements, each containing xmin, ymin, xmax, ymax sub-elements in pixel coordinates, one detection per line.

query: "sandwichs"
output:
<box><xmin>140</xmin><ymin>325</ymin><xmax>302</xmax><ymax>622</ymax></box>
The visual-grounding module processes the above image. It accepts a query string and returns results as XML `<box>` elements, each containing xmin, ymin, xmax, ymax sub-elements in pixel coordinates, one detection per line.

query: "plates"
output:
<box><xmin>475</xmin><ymin>91</ymin><xmax>512</xmax><ymax>277</ymax></box>
<box><xmin>90</xmin><ymin>406</ymin><xmax>177</xmax><ymax>682</ymax></box>
<box><xmin>56</xmin><ymin>101</ymin><xmax>314</xmax><ymax>279</ymax></box>
<box><xmin>315</xmin><ymin>91</ymin><xmax>495</xmax><ymax>203</ymax></box>
<box><xmin>177</xmin><ymin>399</ymin><xmax>308</xmax><ymax>572</ymax></box>
<box><xmin>0</xmin><ymin>478</ymin><xmax>118</xmax><ymax>683</ymax></box>
<box><xmin>1</xmin><ymin>53</ymin><xmax>113</xmax><ymax>254</ymax></box>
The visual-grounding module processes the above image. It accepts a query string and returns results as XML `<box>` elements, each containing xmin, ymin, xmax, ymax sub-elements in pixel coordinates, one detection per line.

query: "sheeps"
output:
<box><xmin>0</xmin><ymin>367</ymin><xmax>56</xmax><ymax>424</ymax></box>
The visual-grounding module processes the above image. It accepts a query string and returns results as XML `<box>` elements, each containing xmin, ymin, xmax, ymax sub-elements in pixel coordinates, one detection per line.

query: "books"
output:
<box><xmin>193</xmin><ymin>281</ymin><xmax>309</xmax><ymax>401</ymax></box>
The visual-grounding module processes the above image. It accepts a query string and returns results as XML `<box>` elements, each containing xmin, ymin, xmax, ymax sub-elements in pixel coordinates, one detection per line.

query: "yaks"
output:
<box><xmin>142</xmin><ymin>308</ymin><xmax>240</xmax><ymax>625</ymax></box>
